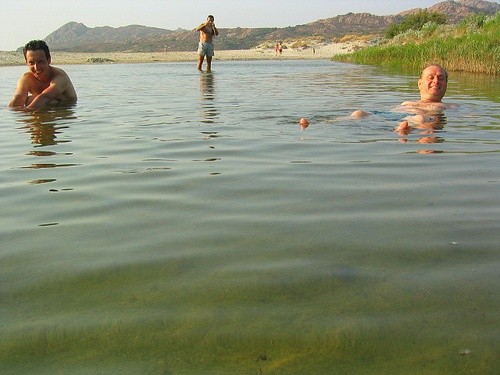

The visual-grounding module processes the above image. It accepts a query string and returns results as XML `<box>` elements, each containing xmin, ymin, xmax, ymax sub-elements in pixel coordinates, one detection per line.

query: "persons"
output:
<box><xmin>196</xmin><ymin>14</ymin><xmax>218</xmax><ymax>73</ymax></box>
<box><xmin>275</xmin><ymin>43</ymin><xmax>279</xmax><ymax>51</ymax></box>
<box><xmin>300</xmin><ymin>63</ymin><xmax>459</xmax><ymax>134</ymax></box>
<box><xmin>7</xmin><ymin>39</ymin><xmax>78</xmax><ymax>111</ymax></box>
<box><xmin>279</xmin><ymin>42</ymin><xmax>283</xmax><ymax>54</ymax></box>
